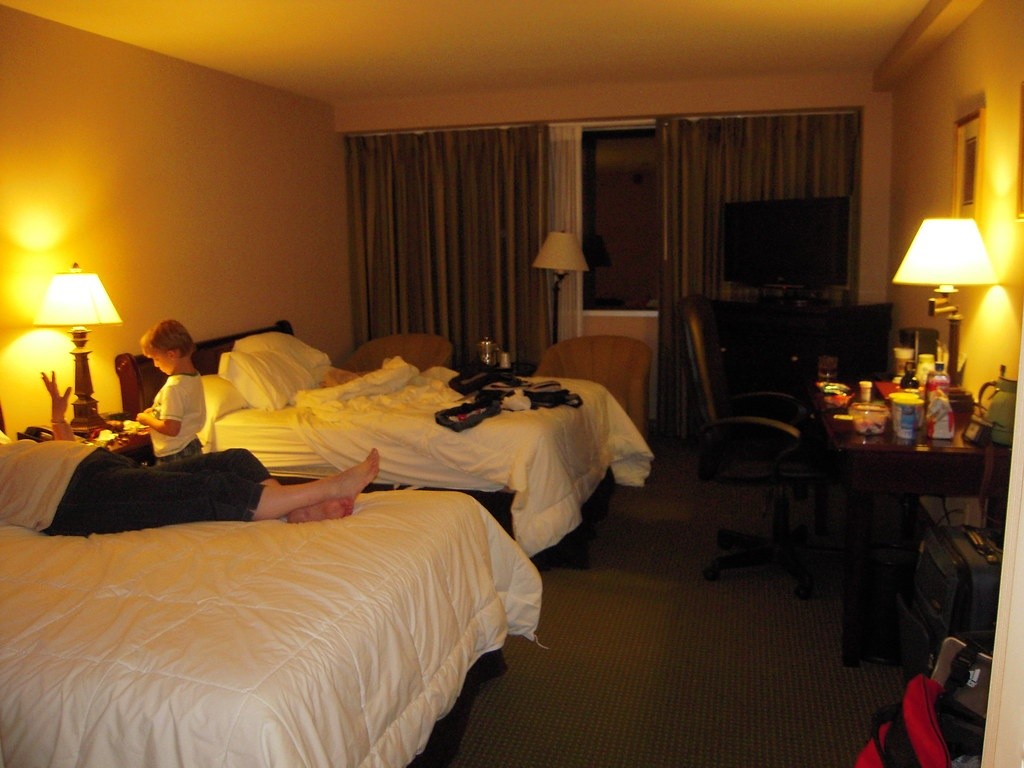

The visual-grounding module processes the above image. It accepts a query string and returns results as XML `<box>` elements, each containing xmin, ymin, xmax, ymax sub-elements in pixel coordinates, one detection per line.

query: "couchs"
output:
<box><xmin>534</xmin><ymin>335</ymin><xmax>651</xmax><ymax>447</ymax></box>
<box><xmin>339</xmin><ymin>335</ymin><xmax>452</xmax><ymax>374</ymax></box>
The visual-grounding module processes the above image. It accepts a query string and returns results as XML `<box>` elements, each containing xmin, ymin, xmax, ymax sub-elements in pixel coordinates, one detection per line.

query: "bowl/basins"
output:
<box><xmin>849</xmin><ymin>402</ymin><xmax>889</xmax><ymax>436</ymax></box>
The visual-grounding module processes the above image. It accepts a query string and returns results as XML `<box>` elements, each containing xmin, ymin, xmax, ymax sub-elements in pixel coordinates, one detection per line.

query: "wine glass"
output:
<box><xmin>817</xmin><ymin>356</ymin><xmax>838</xmax><ymax>382</ymax></box>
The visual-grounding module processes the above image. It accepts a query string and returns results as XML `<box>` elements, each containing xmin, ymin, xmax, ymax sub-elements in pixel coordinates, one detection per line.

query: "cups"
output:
<box><xmin>887</xmin><ymin>392</ymin><xmax>925</xmax><ymax>440</ymax></box>
<box><xmin>894</xmin><ymin>348</ymin><xmax>914</xmax><ymax>376</ymax></box>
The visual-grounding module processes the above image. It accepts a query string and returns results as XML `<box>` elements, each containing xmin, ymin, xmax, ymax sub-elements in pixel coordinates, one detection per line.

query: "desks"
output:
<box><xmin>720</xmin><ymin>293</ymin><xmax>892</xmax><ymax>455</ymax></box>
<box><xmin>459</xmin><ymin>358</ymin><xmax>533</xmax><ymax>379</ymax></box>
<box><xmin>812</xmin><ymin>385</ymin><xmax>985</xmax><ymax>664</ymax></box>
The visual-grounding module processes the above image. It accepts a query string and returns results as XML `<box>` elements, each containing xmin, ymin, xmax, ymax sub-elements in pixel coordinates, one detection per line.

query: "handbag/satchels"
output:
<box><xmin>854</xmin><ymin>669</ymin><xmax>986</xmax><ymax>768</ymax></box>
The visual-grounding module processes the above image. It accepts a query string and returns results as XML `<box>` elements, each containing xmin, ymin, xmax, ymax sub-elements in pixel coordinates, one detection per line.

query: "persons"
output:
<box><xmin>0</xmin><ymin>373</ymin><xmax>378</xmax><ymax>536</ymax></box>
<box><xmin>136</xmin><ymin>319</ymin><xmax>206</xmax><ymax>466</ymax></box>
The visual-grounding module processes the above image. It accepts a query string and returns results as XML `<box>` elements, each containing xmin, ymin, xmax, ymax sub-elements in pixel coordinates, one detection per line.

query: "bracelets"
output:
<box><xmin>50</xmin><ymin>419</ymin><xmax>67</xmax><ymax>424</ymax></box>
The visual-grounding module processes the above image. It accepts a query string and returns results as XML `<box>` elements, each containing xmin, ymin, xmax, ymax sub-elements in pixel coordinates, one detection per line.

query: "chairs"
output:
<box><xmin>678</xmin><ymin>296</ymin><xmax>819</xmax><ymax>600</ymax></box>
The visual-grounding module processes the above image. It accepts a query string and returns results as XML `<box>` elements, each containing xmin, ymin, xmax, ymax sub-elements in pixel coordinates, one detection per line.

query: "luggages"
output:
<box><xmin>896</xmin><ymin>522</ymin><xmax>1003</xmax><ymax>688</ymax></box>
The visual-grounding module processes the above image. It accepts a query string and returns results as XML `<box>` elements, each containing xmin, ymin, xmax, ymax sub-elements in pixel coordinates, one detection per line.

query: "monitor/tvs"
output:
<box><xmin>721</xmin><ymin>195</ymin><xmax>855</xmax><ymax>306</ymax></box>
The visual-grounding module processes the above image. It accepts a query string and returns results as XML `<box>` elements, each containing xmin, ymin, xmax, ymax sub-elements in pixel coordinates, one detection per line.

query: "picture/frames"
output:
<box><xmin>953</xmin><ymin>108</ymin><xmax>986</xmax><ymax>220</ymax></box>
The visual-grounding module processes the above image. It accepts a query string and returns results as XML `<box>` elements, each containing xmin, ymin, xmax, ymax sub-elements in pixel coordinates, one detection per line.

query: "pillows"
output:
<box><xmin>196</xmin><ymin>377</ymin><xmax>246</xmax><ymax>446</ymax></box>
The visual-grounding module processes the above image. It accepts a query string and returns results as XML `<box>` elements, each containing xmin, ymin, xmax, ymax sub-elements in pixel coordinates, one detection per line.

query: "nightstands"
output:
<box><xmin>69</xmin><ymin>422</ymin><xmax>157</xmax><ymax>473</ymax></box>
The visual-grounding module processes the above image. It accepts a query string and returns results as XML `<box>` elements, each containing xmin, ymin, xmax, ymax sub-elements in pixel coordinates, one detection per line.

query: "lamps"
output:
<box><xmin>532</xmin><ymin>233</ymin><xmax>591</xmax><ymax>345</ymax></box>
<box><xmin>37</xmin><ymin>262</ymin><xmax>125</xmax><ymax>435</ymax></box>
<box><xmin>892</xmin><ymin>218</ymin><xmax>996</xmax><ymax>387</ymax></box>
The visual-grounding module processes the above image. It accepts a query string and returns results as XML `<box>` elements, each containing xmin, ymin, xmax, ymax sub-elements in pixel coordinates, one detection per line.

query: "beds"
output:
<box><xmin>114</xmin><ymin>320</ymin><xmax>653</xmax><ymax>572</ymax></box>
<box><xmin>1</xmin><ymin>489</ymin><xmax>543</xmax><ymax>768</ymax></box>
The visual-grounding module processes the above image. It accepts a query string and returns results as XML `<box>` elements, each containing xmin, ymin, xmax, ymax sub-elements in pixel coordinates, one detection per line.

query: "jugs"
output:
<box><xmin>978</xmin><ymin>375</ymin><xmax>1018</xmax><ymax>446</ymax></box>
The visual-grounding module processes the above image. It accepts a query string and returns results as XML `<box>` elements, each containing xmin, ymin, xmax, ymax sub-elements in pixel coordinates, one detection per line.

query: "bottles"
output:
<box><xmin>916</xmin><ymin>354</ymin><xmax>936</xmax><ymax>384</ymax></box>
<box><xmin>900</xmin><ymin>360</ymin><xmax>920</xmax><ymax>393</ymax></box>
<box><xmin>924</xmin><ymin>363</ymin><xmax>950</xmax><ymax>399</ymax></box>
<box><xmin>859</xmin><ymin>380</ymin><xmax>873</xmax><ymax>402</ymax></box>
